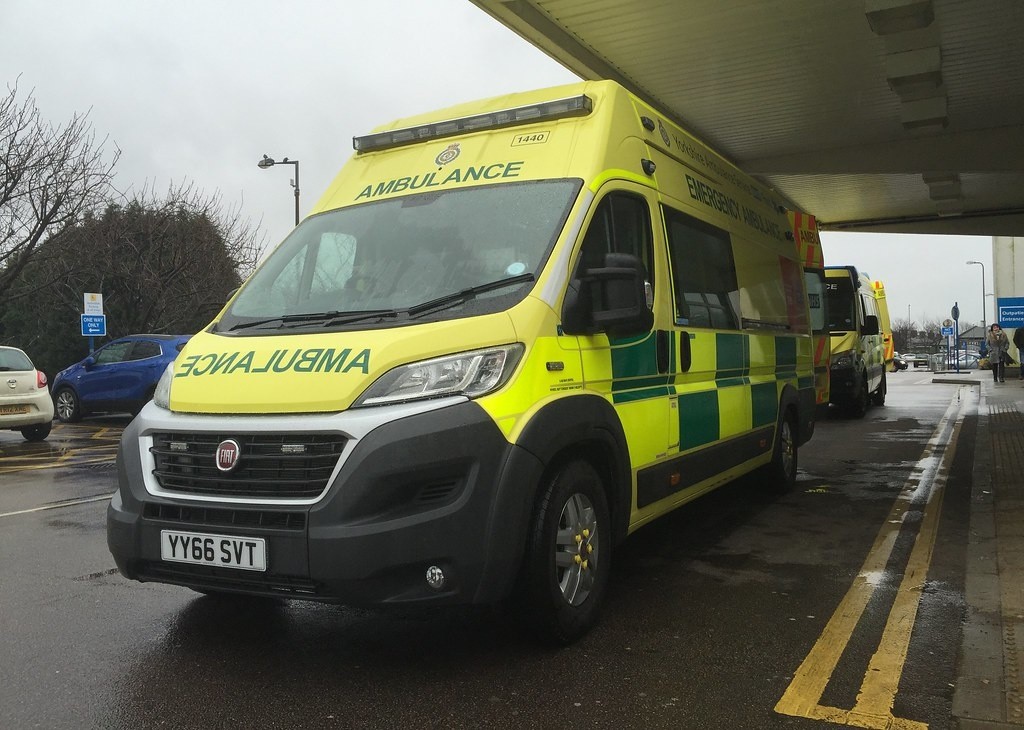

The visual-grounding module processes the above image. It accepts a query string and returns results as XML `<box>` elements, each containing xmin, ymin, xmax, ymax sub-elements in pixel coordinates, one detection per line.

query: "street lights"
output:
<box><xmin>965</xmin><ymin>260</ymin><xmax>987</xmax><ymax>350</ymax></box>
<box><xmin>256</xmin><ymin>157</ymin><xmax>299</xmax><ymax>227</ymax></box>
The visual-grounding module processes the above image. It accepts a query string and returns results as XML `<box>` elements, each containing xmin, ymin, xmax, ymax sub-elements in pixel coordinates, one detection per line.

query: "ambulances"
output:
<box><xmin>105</xmin><ymin>77</ymin><xmax>834</xmax><ymax>650</ymax></box>
<box><xmin>824</xmin><ymin>264</ymin><xmax>896</xmax><ymax>421</ymax></box>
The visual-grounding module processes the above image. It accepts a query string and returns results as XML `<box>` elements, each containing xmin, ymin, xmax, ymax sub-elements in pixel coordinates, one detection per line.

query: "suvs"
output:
<box><xmin>50</xmin><ymin>333</ymin><xmax>198</xmax><ymax>426</ymax></box>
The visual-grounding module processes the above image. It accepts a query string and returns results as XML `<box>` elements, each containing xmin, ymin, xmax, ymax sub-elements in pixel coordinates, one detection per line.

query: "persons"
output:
<box><xmin>984</xmin><ymin>323</ymin><xmax>1010</xmax><ymax>384</ymax></box>
<box><xmin>1013</xmin><ymin>328</ymin><xmax>1024</xmax><ymax>379</ymax></box>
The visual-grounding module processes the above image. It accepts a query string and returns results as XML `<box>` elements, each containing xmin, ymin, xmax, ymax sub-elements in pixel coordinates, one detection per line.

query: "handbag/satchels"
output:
<box><xmin>1003</xmin><ymin>351</ymin><xmax>1016</xmax><ymax>366</ymax></box>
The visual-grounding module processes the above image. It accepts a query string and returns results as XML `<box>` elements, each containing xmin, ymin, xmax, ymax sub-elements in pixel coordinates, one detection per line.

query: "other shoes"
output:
<box><xmin>999</xmin><ymin>379</ymin><xmax>1004</xmax><ymax>382</ymax></box>
<box><xmin>994</xmin><ymin>376</ymin><xmax>997</xmax><ymax>382</ymax></box>
<box><xmin>1019</xmin><ymin>377</ymin><xmax>1023</xmax><ymax>380</ymax></box>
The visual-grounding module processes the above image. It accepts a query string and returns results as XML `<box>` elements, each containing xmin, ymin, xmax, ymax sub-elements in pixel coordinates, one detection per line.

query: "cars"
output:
<box><xmin>890</xmin><ymin>349</ymin><xmax>982</xmax><ymax>373</ymax></box>
<box><xmin>0</xmin><ymin>346</ymin><xmax>54</xmax><ymax>443</ymax></box>
